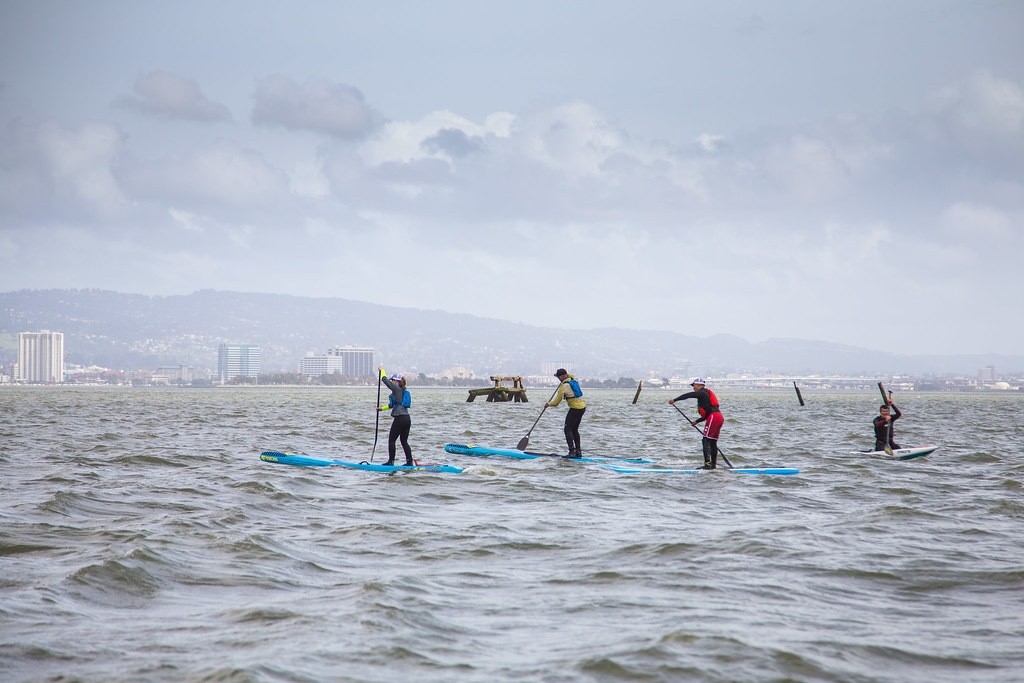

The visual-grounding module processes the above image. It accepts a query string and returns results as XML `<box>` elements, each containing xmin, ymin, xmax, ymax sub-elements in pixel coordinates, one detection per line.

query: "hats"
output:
<box><xmin>554</xmin><ymin>369</ymin><xmax>567</xmax><ymax>376</ymax></box>
<box><xmin>388</xmin><ymin>373</ymin><xmax>403</xmax><ymax>381</ymax></box>
<box><xmin>690</xmin><ymin>378</ymin><xmax>706</xmax><ymax>386</ymax></box>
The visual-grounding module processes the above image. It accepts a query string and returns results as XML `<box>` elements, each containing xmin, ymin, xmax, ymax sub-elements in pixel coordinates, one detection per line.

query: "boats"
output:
<box><xmin>260</xmin><ymin>451</ymin><xmax>465</xmax><ymax>473</ymax></box>
<box><xmin>443</xmin><ymin>443</ymin><xmax>652</xmax><ymax>466</ymax></box>
<box><xmin>850</xmin><ymin>446</ymin><xmax>938</xmax><ymax>462</ymax></box>
<box><xmin>609</xmin><ymin>466</ymin><xmax>801</xmax><ymax>475</ymax></box>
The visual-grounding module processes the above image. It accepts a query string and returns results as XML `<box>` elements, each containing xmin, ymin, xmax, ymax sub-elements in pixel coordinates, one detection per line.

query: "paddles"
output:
<box><xmin>370</xmin><ymin>370</ymin><xmax>382</xmax><ymax>462</ymax></box>
<box><xmin>883</xmin><ymin>390</ymin><xmax>894</xmax><ymax>455</ymax></box>
<box><xmin>672</xmin><ymin>403</ymin><xmax>733</xmax><ymax>468</ymax></box>
<box><xmin>516</xmin><ymin>382</ymin><xmax>561</xmax><ymax>451</ymax></box>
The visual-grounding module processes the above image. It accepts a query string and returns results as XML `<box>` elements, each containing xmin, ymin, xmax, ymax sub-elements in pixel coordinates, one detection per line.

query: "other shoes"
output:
<box><xmin>562</xmin><ymin>452</ymin><xmax>576</xmax><ymax>458</ymax></box>
<box><xmin>575</xmin><ymin>453</ymin><xmax>582</xmax><ymax>459</ymax></box>
<box><xmin>696</xmin><ymin>461</ymin><xmax>716</xmax><ymax>469</ymax></box>
<box><xmin>402</xmin><ymin>463</ymin><xmax>413</xmax><ymax>466</ymax></box>
<box><xmin>382</xmin><ymin>461</ymin><xmax>394</xmax><ymax>466</ymax></box>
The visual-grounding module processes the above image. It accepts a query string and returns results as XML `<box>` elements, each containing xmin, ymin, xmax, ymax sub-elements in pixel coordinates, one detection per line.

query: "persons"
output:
<box><xmin>669</xmin><ymin>378</ymin><xmax>724</xmax><ymax>469</ymax></box>
<box><xmin>873</xmin><ymin>398</ymin><xmax>902</xmax><ymax>451</ymax></box>
<box><xmin>544</xmin><ymin>367</ymin><xmax>586</xmax><ymax>459</ymax></box>
<box><xmin>376</xmin><ymin>365</ymin><xmax>413</xmax><ymax>467</ymax></box>
